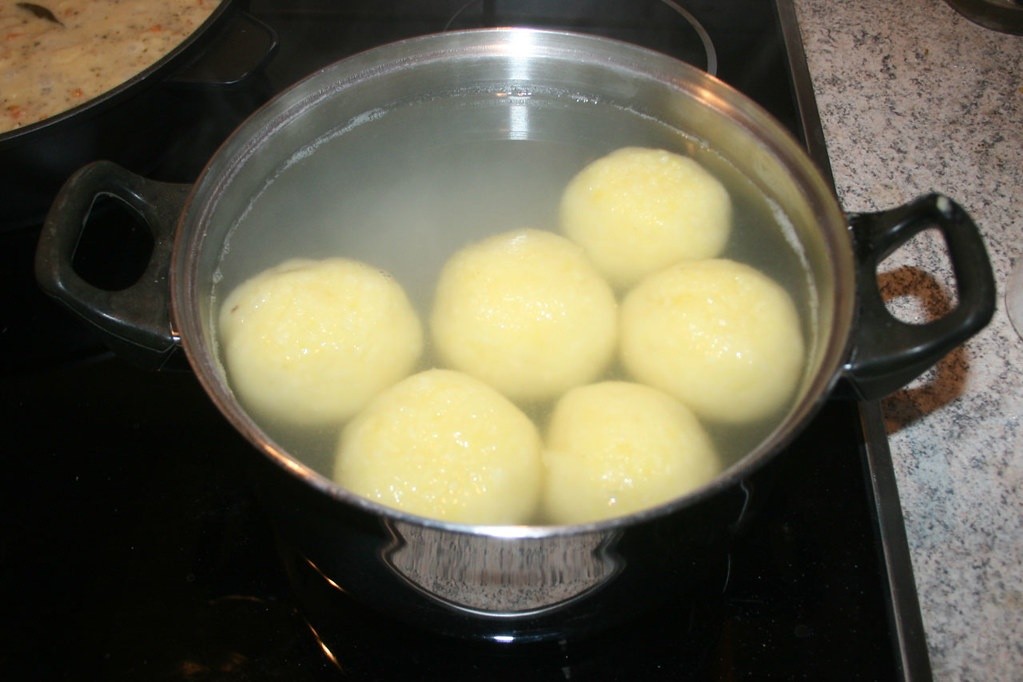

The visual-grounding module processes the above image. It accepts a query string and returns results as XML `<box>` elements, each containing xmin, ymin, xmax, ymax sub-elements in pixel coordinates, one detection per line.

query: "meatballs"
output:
<box><xmin>331</xmin><ymin>369</ymin><xmax>547</xmax><ymax>526</ymax></box>
<box><xmin>220</xmin><ymin>255</ymin><xmax>422</xmax><ymax>431</ymax></box>
<box><xmin>425</xmin><ymin>224</ymin><xmax>620</xmax><ymax>405</ymax></box>
<box><xmin>617</xmin><ymin>257</ymin><xmax>807</xmax><ymax>424</ymax></box>
<box><xmin>555</xmin><ymin>145</ymin><xmax>737</xmax><ymax>288</ymax></box>
<box><xmin>542</xmin><ymin>379</ymin><xmax>719</xmax><ymax>528</ymax></box>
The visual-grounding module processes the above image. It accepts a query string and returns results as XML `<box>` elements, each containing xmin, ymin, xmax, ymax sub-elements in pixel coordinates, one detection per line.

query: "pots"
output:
<box><xmin>38</xmin><ymin>26</ymin><xmax>1003</xmax><ymax>650</ymax></box>
